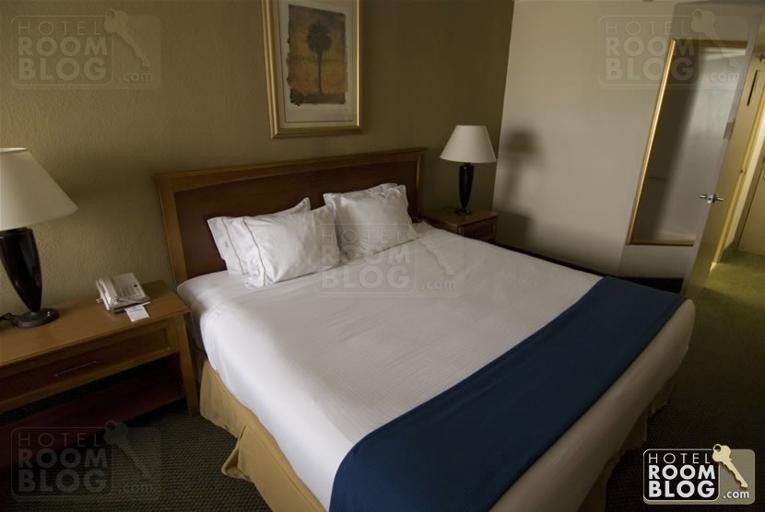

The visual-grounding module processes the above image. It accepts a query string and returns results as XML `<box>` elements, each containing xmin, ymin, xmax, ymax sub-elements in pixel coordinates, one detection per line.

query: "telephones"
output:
<box><xmin>96</xmin><ymin>272</ymin><xmax>146</xmax><ymax>311</ymax></box>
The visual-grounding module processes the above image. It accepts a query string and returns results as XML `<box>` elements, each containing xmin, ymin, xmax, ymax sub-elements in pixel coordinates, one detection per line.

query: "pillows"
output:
<box><xmin>203</xmin><ymin>198</ymin><xmax>313</xmax><ymax>276</ymax></box>
<box><xmin>321</xmin><ymin>183</ymin><xmax>397</xmax><ymax>208</ymax></box>
<box><xmin>331</xmin><ymin>185</ymin><xmax>421</xmax><ymax>258</ymax></box>
<box><xmin>231</xmin><ymin>206</ymin><xmax>345</xmax><ymax>288</ymax></box>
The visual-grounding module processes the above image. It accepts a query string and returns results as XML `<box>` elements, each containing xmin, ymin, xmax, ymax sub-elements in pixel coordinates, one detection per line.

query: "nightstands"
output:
<box><xmin>433</xmin><ymin>207</ymin><xmax>498</xmax><ymax>243</ymax></box>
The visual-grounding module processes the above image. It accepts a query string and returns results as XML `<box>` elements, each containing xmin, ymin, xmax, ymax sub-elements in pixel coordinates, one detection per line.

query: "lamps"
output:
<box><xmin>437</xmin><ymin>120</ymin><xmax>498</xmax><ymax>215</ymax></box>
<box><xmin>0</xmin><ymin>143</ymin><xmax>80</xmax><ymax>329</ymax></box>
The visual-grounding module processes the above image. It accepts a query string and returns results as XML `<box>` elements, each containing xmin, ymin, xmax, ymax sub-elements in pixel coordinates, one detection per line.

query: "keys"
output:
<box><xmin>103</xmin><ymin>419</ymin><xmax>151</xmax><ymax>481</ymax></box>
<box><xmin>712</xmin><ymin>443</ymin><xmax>748</xmax><ymax>488</ymax></box>
<box><xmin>691</xmin><ymin>8</ymin><xmax>738</xmax><ymax>68</ymax></box>
<box><xmin>103</xmin><ymin>7</ymin><xmax>152</xmax><ymax>69</ymax></box>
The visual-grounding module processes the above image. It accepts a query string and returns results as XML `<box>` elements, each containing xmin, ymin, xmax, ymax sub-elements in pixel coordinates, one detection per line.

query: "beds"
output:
<box><xmin>146</xmin><ymin>144</ymin><xmax>698</xmax><ymax>509</ymax></box>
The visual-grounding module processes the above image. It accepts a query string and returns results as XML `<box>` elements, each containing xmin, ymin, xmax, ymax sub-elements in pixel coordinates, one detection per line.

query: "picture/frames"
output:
<box><xmin>262</xmin><ymin>1</ymin><xmax>366</xmax><ymax>140</ymax></box>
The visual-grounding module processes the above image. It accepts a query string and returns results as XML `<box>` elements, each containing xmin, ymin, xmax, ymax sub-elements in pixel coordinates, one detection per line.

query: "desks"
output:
<box><xmin>0</xmin><ymin>278</ymin><xmax>200</xmax><ymax>475</ymax></box>
